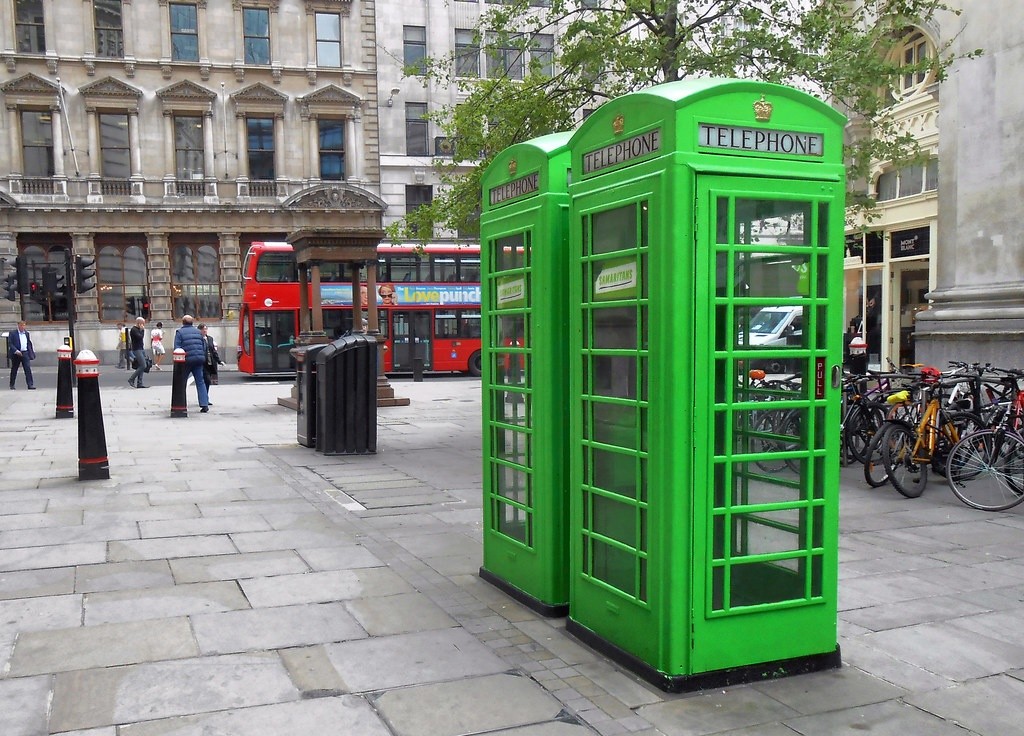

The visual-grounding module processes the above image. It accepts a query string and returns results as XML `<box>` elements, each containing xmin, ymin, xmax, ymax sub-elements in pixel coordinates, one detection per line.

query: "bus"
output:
<box><xmin>225</xmin><ymin>241</ymin><xmax>525</xmax><ymax>377</ymax></box>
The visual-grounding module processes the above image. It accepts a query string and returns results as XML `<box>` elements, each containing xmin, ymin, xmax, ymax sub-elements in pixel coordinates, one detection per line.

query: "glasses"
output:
<box><xmin>380</xmin><ymin>293</ymin><xmax>393</xmax><ymax>299</ymax></box>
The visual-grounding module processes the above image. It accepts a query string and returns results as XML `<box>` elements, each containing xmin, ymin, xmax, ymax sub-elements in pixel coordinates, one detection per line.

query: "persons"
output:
<box><xmin>174</xmin><ymin>315</ymin><xmax>209</xmax><ymax>412</ymax></box>
<box><xmin>360</xmin><ymin>286</ymin><xmax>367</xmax><ymax>306</ymax></box>
<box><xmin>378</xmin><ymin>285</ymin><xmax>398</xmax><ymax>305</ymax></box>
<box><xmin>196</xmin><ymin>323</ymin><xmax>225</xmax><ymax>405</ymax></box>
<box><xmin>115</xmin><ymin>323</ymin><xmax>135</xmax><ymax>369</ymax></box>
<box><xmin>244</xmin><ymin>330</ymin><xmax>250</xmax><ymax>355</ymax></box>
<box><xmin>151</xmin><ymin>322</ymin><xmax>165</xmax><ymax>370</ymax></box>
<box><xmin>127</xmin><ymin>317</ymin><xmax>152</xmax><ymax>388</ymax></box>
<box><xmin>340</xmin><ymin>318</ymin><xmax>380</xmax><ymax>371</ymax></box>
<box><xmin>8</xmin><ymin>321</ymin><xmax>36</xmax><ymax>389</ymax></box>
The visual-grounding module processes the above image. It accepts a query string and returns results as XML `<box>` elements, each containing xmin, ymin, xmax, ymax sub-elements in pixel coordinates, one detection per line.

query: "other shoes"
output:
<box><xmin>155</xmin><ymin>367</ymin><xmax>158</xmax><ymax>369</ymax></box>
<box><xmin>200</xmin><ymin>407</ymin><xmax>209</xmax><ymax>413</ymax></box>
<box><xmin>208</xmin><ymin>402</ymin><xmax>213</xmax><ymax>406</ymax></box>
<box><xmin>128</xmin><ymin>378</ymin><xmax>137</xmax><ymax>388</ymax></box>
<box><xmin>10</xmin><ymin>387</ymin><xmax>16</xmax><ymax>390</ymax></box>
<box><xmin>137</xmin><ymin>384</ymin><xmax>150</xmax><ymax>388</ymax></box>
<box><xmin>28</xmin><ymin>384</ymin><xmax>36</xmax><ymax>389</ymax></box>
<box><xmin>155</xmin><ymin>364</ymin><xmax>162</xmax><ymax>371</ymax></box>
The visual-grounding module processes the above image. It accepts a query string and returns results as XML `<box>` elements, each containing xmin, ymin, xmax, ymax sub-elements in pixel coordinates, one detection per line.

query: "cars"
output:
<box><xmin>738</xmin><ymin>306</ymin><xmax>804</xmax><ymax>374</ymax></box>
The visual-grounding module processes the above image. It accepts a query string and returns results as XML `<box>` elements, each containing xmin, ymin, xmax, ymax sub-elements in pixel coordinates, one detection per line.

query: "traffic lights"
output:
<box><xmin>50</xmin><ymin>267</ymin><xmax>65</xmax><ymax>302</ymax></box>
<box><xmin>142</xmin><ymin>296</ymin><xmax>152</xmax><ymax>320</ymax></box>
<box><xmin>76</xmin><ymin>254</ymin><xmax>96</xmax><ymax>294</ymax></box>
<box><xmin>127</xmin><ymin>296</ymin><xmax>136</xmax><ymax>315</ymax></box>
<box><xmin>5</xmin><ymin>255</ymin><xmax>20</xmax><ymax>301</ymax></box>
<box><xmin>30</xmin><ymin>282</ymin><xmax>41</xmax><ymax>301</ymax></box>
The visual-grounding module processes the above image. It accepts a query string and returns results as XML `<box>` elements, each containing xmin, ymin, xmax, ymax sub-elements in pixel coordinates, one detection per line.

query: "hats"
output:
<box><xmin>156</xmin><ymin>322</ymin><xmax>162</xmax><ymax>328</ymax></box>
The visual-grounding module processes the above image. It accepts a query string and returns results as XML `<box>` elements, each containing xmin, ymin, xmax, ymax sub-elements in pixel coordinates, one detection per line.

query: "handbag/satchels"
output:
<box><xmin>132</xmin><ymin>355</ymin><xmax>153</xmax><ymax>374</ymax></box>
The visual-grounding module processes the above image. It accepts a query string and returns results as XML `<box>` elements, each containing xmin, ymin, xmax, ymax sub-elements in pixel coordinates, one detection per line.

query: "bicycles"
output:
<box><xmin>733</xmin><ymin>357</ymin><xmax>1024</xmax><ymax>511</ymax></box>
